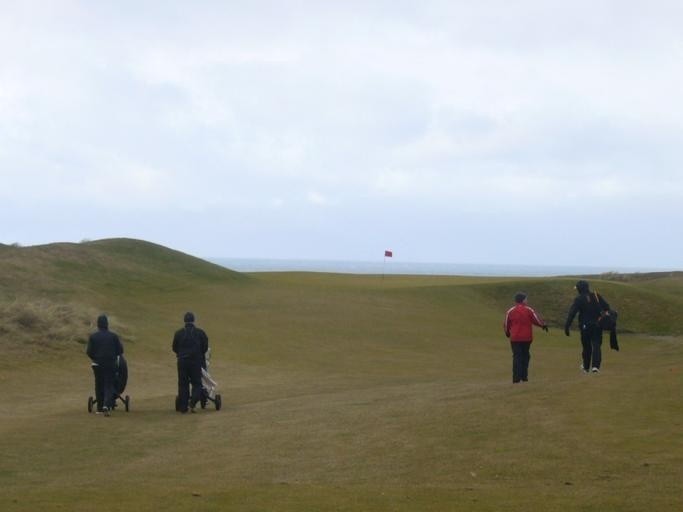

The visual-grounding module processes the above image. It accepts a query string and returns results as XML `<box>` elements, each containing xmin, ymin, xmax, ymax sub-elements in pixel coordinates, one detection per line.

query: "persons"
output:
<box><xmin>503</xmin><ymin>291</ymin><xmax>549</xmax><ymax>385</ymax></box>
<box><xmin>172</xmin><ymin>312</ymin><xmax>209</xmax><ymax>414</ymax></box>
<box><xmin>564</xmin><ymin>279</ymin><xmax>611</xmax><ymax>375</ymax></box>
<box><xmin>85</xmin><ymin>315</ymin><xmax>125</xmax><ymax>418</ymax></box>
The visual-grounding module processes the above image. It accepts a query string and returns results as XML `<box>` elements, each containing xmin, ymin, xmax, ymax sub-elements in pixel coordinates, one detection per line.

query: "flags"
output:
<box><xmin>385</xmin><ymin>247</ymin><xmax>393</xmax><ymax>257</ymax></box>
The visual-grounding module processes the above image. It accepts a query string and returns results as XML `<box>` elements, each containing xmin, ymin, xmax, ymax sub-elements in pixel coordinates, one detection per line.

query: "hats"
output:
<box><xmin>515</xmin><ymin>292</ymin><xmax>526</xmax><ymax>303</ymax></box>
<box><xmin>98</xmin><ymin>315</ymin><xmax>108</xmax><ymax>328</ymax></box>
<box><xmin>184</xmin><ymin>312</ymin><xmax>194</xmax><ymax>322</ymax></box>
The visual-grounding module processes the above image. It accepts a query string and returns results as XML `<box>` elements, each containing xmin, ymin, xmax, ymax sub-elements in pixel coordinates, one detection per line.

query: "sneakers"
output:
<box><xmin>96</xmin><ymin>406</ymin><xmax>111</xmax><ymax>416</ymax></box>
<box><xmin>190</xmin><ymin>401</ymin><xmax>196</xmax><ymax>412</ymax></box>
<box><xmin>580</xmin><ymin>363</ymin><xmax>601</xmax><ymax>375</ymax></box>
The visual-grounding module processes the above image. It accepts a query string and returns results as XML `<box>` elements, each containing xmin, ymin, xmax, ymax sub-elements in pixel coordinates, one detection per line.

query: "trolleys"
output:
<box><xmin>87</xmin><ymin>355</ymin><xmax>130</xmax><ymax>415</ymax></box>
<box><xmin>175</xmin><ymin>348</ymin><xmax>221</xmax><ymax>412</ymax></box>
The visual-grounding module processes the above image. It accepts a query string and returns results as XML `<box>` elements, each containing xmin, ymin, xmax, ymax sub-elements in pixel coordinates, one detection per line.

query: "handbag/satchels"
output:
<box><xmin>598</xmin><ymin>309</ymin><xmax>617</xmax><ymax>331</ymax></box>
